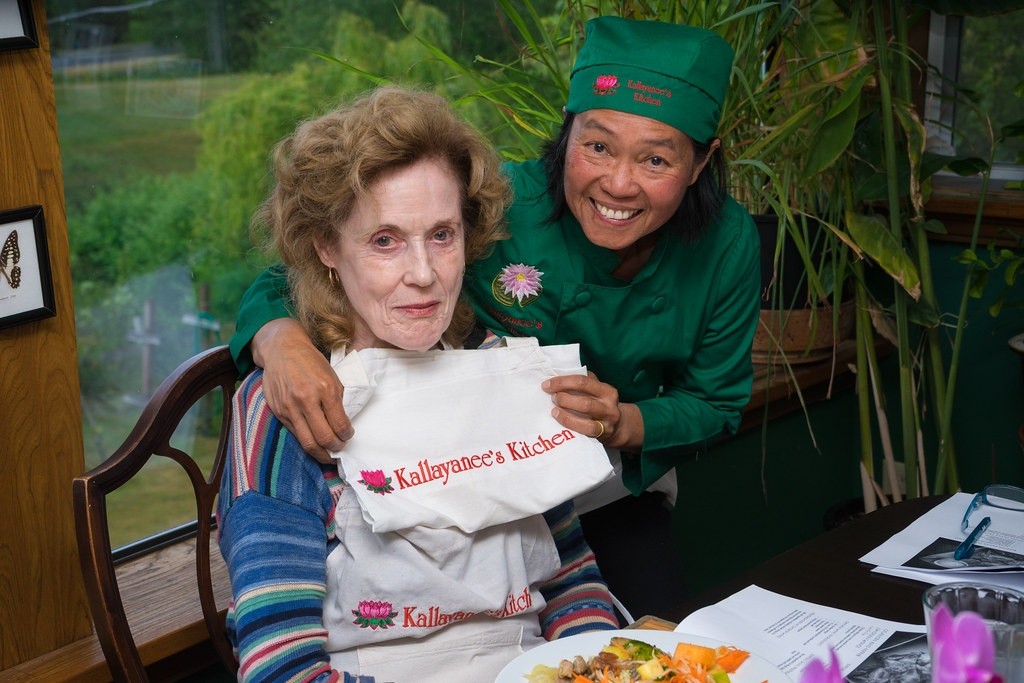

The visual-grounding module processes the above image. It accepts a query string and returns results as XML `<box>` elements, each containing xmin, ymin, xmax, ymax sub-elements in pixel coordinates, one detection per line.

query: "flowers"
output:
<box><xmin>799</xmin><ymin>602</ymin><xmax>1006</xmax><ymax>682</ymax></box>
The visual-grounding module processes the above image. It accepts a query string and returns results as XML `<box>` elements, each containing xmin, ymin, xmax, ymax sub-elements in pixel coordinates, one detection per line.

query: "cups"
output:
<box><xmin>921</xmin><ymin>581</ymin><xmax>1024</xmax><ymax>683</ymax></box>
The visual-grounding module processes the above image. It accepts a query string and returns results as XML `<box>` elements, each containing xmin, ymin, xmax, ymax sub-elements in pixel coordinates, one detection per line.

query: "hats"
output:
<box><xmin>566</xmin><ymin>15</ymin><xmax>734</xmax><ymax>144</ymax></box>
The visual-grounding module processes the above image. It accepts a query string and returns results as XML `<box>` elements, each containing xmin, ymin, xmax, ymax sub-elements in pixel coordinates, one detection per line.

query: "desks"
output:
<box><xmin>671</xmin><ymin>495</ymin><xmax>953</xmax><ymax>624</ymax></box>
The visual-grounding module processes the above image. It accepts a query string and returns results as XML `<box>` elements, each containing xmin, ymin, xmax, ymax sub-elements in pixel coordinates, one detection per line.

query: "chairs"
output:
<box><xmin>72</xmin><ymin>345</ymin><xmax>238</xmax><ymax>683</ymax></box>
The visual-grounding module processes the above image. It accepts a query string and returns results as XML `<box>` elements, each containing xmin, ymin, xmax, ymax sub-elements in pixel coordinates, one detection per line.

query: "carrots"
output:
<box><xmin>571</xmin><ymin>642</ymin><xmax>751</xmax><ymax>683</ymax></box>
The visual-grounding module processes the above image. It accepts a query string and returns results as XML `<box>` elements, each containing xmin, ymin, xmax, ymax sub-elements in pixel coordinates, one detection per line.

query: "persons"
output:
<box><xmin>225</xmin><ymin>14</ymin><xmax>761</xmax><ymax>624</ymax></box>
<box><xmin>214</xmin><ymin>84</ymin><xmax>618</xmax><ymax>683</ymax></box>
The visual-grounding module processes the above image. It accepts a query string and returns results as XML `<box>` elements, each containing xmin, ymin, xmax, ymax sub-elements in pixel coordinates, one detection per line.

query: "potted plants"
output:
<box><xmin>276</xmin><ymin>0</ymin><xmax>929</xmax><ymax>494</ymax></box>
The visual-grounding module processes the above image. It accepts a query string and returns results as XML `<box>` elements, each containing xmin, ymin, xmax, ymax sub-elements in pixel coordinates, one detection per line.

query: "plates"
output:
<box><xmin>494</xmin><ymin>628</ymin><xmax>794</xmax><ymax>683</ymax></box>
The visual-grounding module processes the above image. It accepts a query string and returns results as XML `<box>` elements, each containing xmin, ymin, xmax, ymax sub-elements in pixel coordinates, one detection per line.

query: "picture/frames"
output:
<box><xmin>0</xmin><ymin>0</ymin><xmax>39</xmax><ymax>52</ymax></box>
<box><xmin>0</xmin><ymin>204</ymin><xmax>57</xmax><ymax>330</ymax></box>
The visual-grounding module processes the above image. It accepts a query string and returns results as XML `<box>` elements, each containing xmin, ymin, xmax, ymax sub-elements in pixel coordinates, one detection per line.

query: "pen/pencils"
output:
<box><xmin>954</xmin><ymin>517</ymin><xmax>992</xmax><ymax>561</ymax></box>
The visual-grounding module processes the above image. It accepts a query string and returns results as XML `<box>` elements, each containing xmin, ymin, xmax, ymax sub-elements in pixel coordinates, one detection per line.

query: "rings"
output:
<box><xmin>594</xmin><ymin>419</ymin><xmax>604</xmax><ymax>439</ymax></box>
